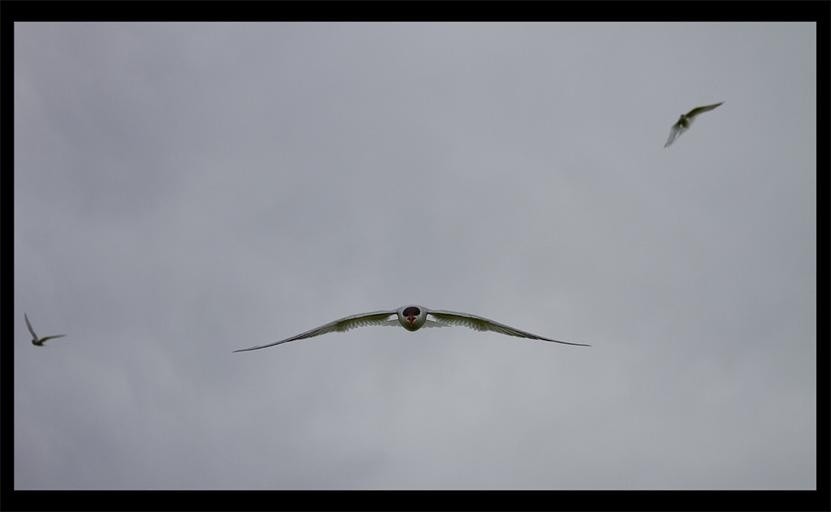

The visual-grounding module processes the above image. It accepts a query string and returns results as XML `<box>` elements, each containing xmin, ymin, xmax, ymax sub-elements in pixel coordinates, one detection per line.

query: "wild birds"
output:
<box><xmin>664</xmin><ymin>102</ymin><xmax>726</xmax><ymax>148</ymax></box>
<box><xmin>231</xmin><ymin>303</ymin><xmax>591</xmax><ymax>354</ymax></box>
<box><xmin>24</xmin><ymin>313</ymin><xmax>66</xmax><ymax>346</ymax></box>
